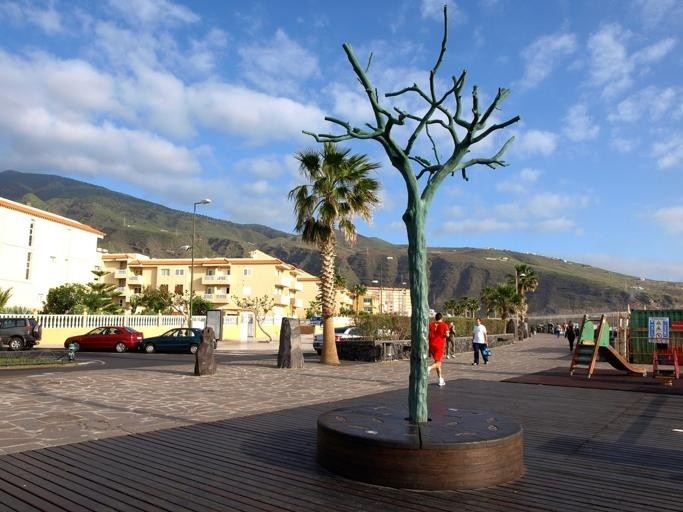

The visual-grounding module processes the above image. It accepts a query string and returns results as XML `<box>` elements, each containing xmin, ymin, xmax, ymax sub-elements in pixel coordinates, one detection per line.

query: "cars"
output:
<box><xmin>64</xmin><ymin>326</ymin><xmax>143</xmax><ymax>353</ymax></box>
<box><xmin>138</xmin><ymin>328</ymin><xmax>217</xmax><ymax>354</ymax></box>
<box><xmin>312</xmin><ymin>326</ymin><xmax>374</xmax><ymax>356</ymax></box>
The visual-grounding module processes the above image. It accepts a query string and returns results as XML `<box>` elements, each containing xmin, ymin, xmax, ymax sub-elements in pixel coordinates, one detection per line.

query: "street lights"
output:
<box><xmin>357</xmin><ymin>278</ymin><xmax>379</xmax><ymax>309</ymax></box>
<box><xmin>380</xmin><ymin>256</ymin><xmax>394</xmax><ymax>314</ymax></box>
<box><xmin>189</xmin><ymin>199</ymin><xmax>212</xmax><ymax>328</ymax></box>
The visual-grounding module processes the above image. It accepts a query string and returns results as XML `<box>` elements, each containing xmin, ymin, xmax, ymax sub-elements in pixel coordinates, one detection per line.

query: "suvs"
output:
<box><xmin>0</xmin><ymin>318</ymin><xmax>42</xmax><ymax>351</ymax></box>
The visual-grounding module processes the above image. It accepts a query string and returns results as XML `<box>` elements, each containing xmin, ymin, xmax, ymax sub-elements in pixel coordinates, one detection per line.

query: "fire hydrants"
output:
<box><xmin>68</xmin><ymin>343</ymin><xmax>77</xmax><ymax>362</ymax></box>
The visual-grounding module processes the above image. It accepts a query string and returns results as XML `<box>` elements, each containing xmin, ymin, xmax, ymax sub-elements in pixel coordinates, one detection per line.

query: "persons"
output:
<box><xmin>445</xmin><ymin>321</ymin><xmax>456</xmax><ymax>359</ymax></box>
<box><xmin>428</xmin><ymin>312</ymin><xmax>446</xmax><ymax>386</ymax></box>
<box><xmin>530</xmin><ymin>320</ymin><xmax>579</xmax><ymax>351</ymax></box>
<box><xmin>472</xmin><ymin>318</ymin><xmax>489</xmax><ymax>365</ymax></box>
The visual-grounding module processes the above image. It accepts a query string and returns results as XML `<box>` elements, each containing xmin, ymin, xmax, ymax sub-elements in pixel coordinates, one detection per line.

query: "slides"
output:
<box><xmin>599</xmin><ymin>344</ymin><xmax>646</xmax><ymax>373</ymax></box>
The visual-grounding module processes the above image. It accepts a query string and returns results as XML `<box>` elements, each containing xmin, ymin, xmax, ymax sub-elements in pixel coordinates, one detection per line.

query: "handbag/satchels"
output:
<box><xmin>481</xmin><ymin>346</ymin><xmax>491</xmax><ymax>356</ymax></box>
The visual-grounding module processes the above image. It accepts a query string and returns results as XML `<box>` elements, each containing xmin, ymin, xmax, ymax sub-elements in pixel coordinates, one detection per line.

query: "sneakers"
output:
<box><xmin>451</xmin><ymin>355</ymin><xmax>455</xmax><ymax>358</ymax></box>
<box><xmin>446</xmin><ymin>355</ymin><xmax>449</xmax><ymax>359</ymax></box>
<box><xmin>484</xmin><ymin>360</ymin><xmax>488</xmax><ymax>364</ymax></box>
<box><xmin>427</xmin><ymin>366</ymin><xmax>431</xmax><ymax>377</ymax></box>
<box><xmin>472</xmin><ymin>362</ymin><xmax>478</xmax><ymax>365</ymax></box>
<box><xmin>437</xmin><ymin>380</ymin><xmax>445</xmax><ymax>386</ymax></box>
